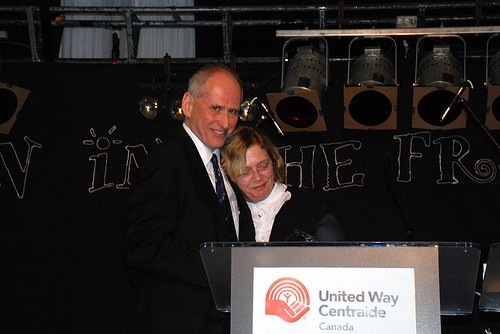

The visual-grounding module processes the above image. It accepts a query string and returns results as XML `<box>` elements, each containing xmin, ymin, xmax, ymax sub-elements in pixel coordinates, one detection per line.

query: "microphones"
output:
<box><xmin>438</xmin><ymin>81</ymin><xmax>469</xmax><ymax>124</ymax></box>
<box><xmin>257</xmin><ymin>98</ymin><xmax>284</xmax><ymax>136</ymax></box>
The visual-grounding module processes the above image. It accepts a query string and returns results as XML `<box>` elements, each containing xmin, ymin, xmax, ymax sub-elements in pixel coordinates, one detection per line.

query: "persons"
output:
<box><xmin>117</xmin><ymin>62</ymin><xmax>254</xmax><ymax>334</ymax></box>
<box><xmin>222</xmin><ymin>126</ymin><xmax>348</xmax><ymax>247</ymax></box>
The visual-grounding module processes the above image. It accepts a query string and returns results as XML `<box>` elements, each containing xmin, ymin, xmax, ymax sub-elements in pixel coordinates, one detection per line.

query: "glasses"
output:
<box><xmin>235</xmin><ymin>159</ymin><xmax>273</xmax><ymax>178</ymax></box>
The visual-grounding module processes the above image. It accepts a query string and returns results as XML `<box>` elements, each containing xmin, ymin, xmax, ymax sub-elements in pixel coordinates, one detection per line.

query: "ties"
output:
<box><xmin>210</xmin><ymin>154</ymin><xmax>238</xmax><ymax>243</ymax></box>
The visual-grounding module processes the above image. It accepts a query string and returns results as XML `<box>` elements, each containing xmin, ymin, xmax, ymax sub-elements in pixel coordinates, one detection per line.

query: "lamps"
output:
<box><xmin>343</xmin><ymin>36</ymin><xmax>400</xmax><ymax>130</ymax></box>
<box><xmin>483</xmin><ymin>33</ymin><xmax>500</xmax><ymax>130</ymax></box>
<box><xmin>266</xmin><ymin>37</ymin><xmax>330</xmax><ymax>134</ymax></box>
<box><xmin>412</xmin><ymin>34</ymin><xmax>470</xmax><ymax>131</ymax></box>
